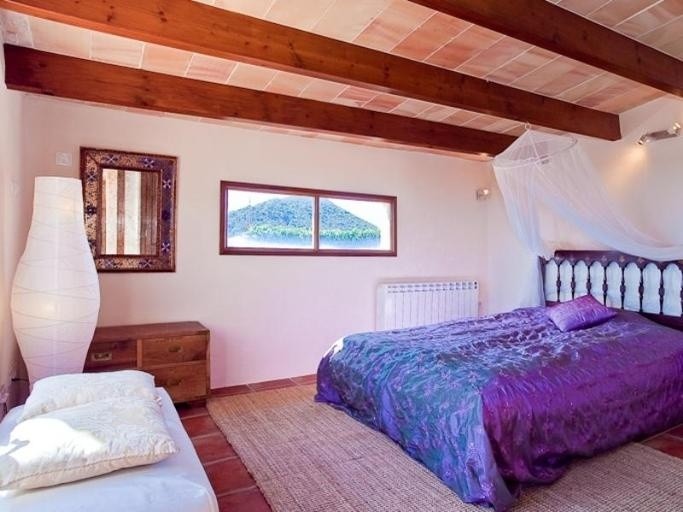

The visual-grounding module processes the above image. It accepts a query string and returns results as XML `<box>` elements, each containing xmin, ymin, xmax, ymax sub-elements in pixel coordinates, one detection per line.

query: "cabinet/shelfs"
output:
<box><xmin>20</xmin><ymin>320</ymin><xmax>212</xmax><ymax>408</ymax></box>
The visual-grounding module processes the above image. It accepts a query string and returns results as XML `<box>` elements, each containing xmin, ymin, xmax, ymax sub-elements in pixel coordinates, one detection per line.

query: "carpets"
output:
<box><xmin>204</xmin><ymin>383</ymin><xmax>683</xmax><ymax>512</ymax></box>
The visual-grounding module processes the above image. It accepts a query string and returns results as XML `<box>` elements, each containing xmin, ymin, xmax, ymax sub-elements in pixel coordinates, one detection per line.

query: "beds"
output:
<box><xmin>315</xmin><ymin>251</ymin><xmax>683</xmax><ymax>512</ymax></box>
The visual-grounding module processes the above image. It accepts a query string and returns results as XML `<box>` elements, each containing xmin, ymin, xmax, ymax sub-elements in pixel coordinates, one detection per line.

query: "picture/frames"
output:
<box><xmin>79</xmin><ymin>145</ymin><xmax>179</xmax><ymax>274</ymax></box>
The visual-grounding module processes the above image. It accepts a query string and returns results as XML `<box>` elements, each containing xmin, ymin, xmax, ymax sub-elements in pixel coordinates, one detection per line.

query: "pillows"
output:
<box><xmin>0</xmin><ymin>370</ymin><xmax>181</xmax><ymax>493</ymax></box>
<box><xmin>544</xmin><ymin>296</ymin><xmax>620</xmax><ymax>333</ymax></box>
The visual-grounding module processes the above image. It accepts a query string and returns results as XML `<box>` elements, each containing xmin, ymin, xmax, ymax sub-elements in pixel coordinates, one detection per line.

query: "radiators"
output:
<box><xmin>377</xmin><ymin>281</ymin><xmax>481</xmax><ymax>331</ymax></box>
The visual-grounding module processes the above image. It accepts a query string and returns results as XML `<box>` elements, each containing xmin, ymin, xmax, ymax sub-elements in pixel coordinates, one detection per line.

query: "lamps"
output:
<box><xmin>637</xmin><ymin>122</ymin><xmax>680</xmax><ymax>146</ymax></box>
<box><xmin>9</xmin><ymin>177</ymin><xmax>100</xmax><ymax>396</ymax></box>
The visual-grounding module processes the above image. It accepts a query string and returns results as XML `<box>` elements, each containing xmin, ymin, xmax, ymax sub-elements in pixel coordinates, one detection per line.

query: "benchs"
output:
<box><xmin>0</xmin><ymin>387</ymin><xmax>218</xmax><ymax>512</ymax></box>
